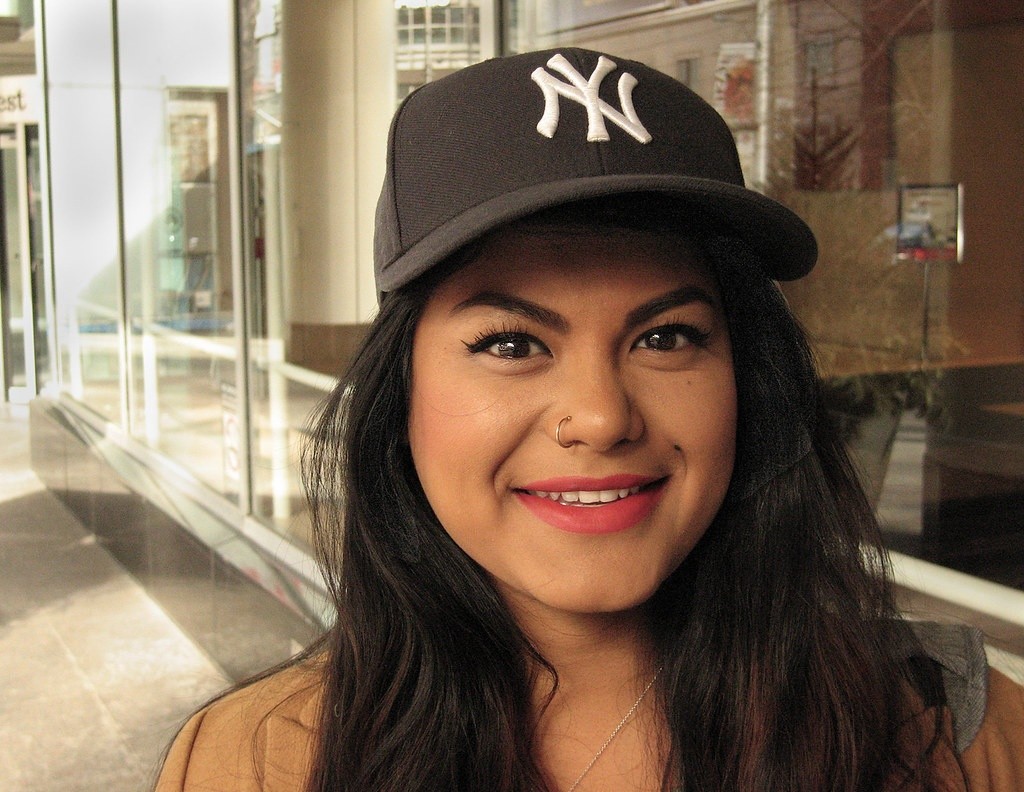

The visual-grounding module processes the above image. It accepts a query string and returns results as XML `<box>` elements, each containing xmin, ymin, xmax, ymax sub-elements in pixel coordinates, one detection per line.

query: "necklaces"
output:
<box><xmin>569</xmin><ymin>660</ymin><xmax>665</xmax><ymax>792</ymax></box>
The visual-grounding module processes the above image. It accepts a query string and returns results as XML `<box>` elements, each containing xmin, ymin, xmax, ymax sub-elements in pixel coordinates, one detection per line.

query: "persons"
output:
<box><xmin>149</xmin><ymin>47</ymin><xmax>1024</xmax><ymax>792</ymax></box>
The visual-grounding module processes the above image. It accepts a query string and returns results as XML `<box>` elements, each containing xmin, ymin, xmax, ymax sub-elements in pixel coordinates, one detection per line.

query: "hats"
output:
<box><xmin>373</xmin><ymin>47</ymin><xmax>821</xmax><ymax>312</ymax></box>
